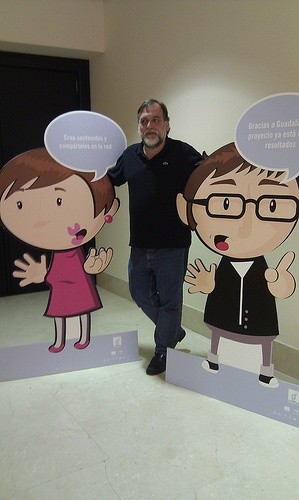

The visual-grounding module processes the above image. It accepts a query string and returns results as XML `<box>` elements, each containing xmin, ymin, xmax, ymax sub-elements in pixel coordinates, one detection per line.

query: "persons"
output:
<box><xmin>105</xmin><ymin>97</ymin><xmax>206</xmax><ymax>377</ymax></box>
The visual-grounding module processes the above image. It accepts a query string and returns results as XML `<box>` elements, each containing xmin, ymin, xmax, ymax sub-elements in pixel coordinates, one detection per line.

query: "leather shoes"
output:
<box><xmin>145</xmin><ymin>351</ymin><xmax>166</xmax><ymax>375</ymax></box>
<box><xmin>173</xmin><ymin>327</ymin><xmax>186</xmax><ymax>349</ymax></box>
